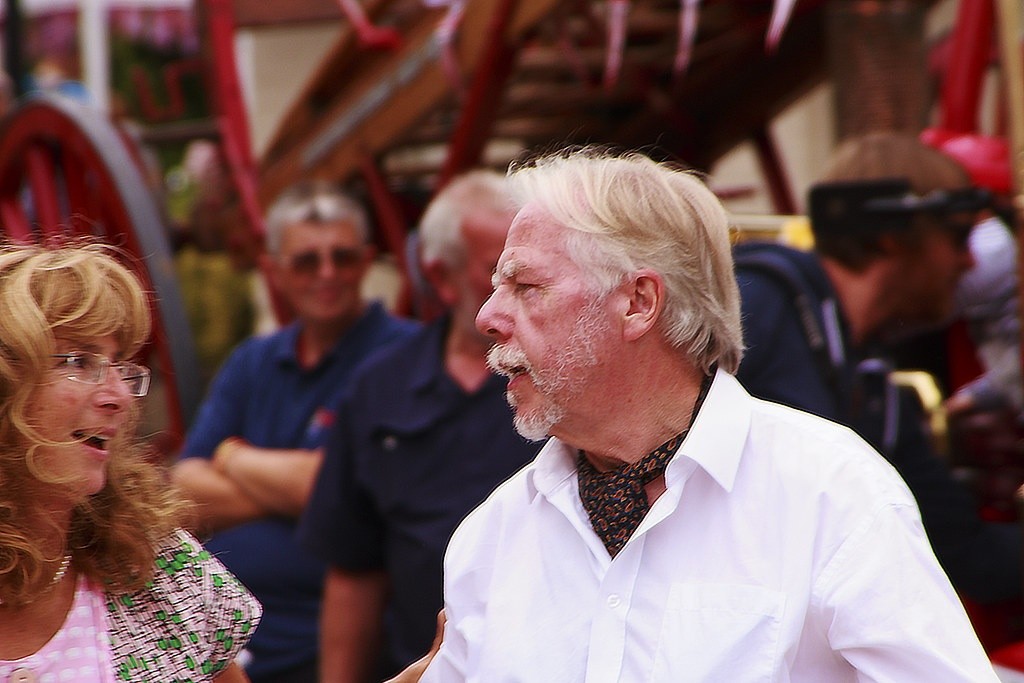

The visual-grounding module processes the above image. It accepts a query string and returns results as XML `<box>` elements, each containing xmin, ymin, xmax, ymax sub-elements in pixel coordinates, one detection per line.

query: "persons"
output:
<box><xmin>419</xmin><ymin>142</ymin><xmax>1000</xmax><ymax>683</ymax></box>
<box><xmin>300</xmin><ymin>171</ymin><xmax>548</xmax><ymax>681</ymax></box>
<box><xmin>0</xmin><ymin>232</ymin><xmax>446</xmax><ymax>682</ymax></box>
<box><xmin>733</xmin><ymin>131</ymin><xmax>1024</xmax><ymax>617</ymax></box>
<box><xmin>172</xmin><ymin>178</ymin><xmax>428</xmax><ymax>681</ymax></box>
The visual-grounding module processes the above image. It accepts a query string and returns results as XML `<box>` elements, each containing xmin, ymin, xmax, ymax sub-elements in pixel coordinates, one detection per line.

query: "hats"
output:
<box><xmin>919</xmin><ymin>127</ymin><xmax>1015</xmax><ymax>192</ymax></box>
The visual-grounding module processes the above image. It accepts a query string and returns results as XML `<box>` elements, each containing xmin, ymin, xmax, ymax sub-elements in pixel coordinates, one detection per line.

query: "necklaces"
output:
<box><xmin>0</xmin><ymin>550</ymin><xmax>72</xmax><ymax>607</ymax></box>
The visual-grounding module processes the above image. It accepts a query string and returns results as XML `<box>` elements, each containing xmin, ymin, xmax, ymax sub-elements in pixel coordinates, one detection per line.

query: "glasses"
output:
<box><xmin>274</xmin><ymin>244</ymin><xmax>366</xmax><ymax>274</ymax></box>
<box><xmin>8</xmin><ymin>350</ymin><xmax>151</xmax><ymax>398</ymax></box>
